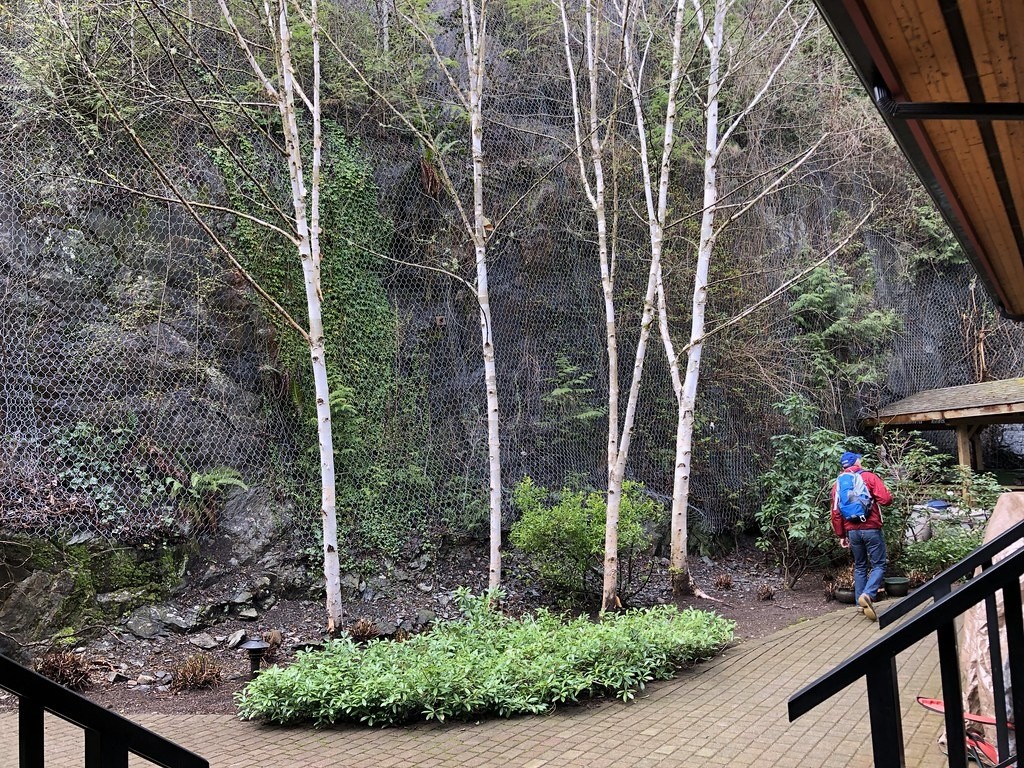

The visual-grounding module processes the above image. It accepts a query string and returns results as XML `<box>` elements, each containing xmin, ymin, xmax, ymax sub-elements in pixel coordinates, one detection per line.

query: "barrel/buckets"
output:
<box><xmin>883</xmin><ymin>576</ymin><xmax>910</xmax><ymax>597</ymax></box>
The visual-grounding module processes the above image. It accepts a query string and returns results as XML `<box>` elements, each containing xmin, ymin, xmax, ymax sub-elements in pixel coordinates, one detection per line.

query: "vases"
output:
<box><xmin>885</xmin><ymin>577</ymin><xmax>909</xmax><ymax>597</ymax></box>
<box><xmin>834</xmin><ymin>584</ymin><xmax>855</xmax><ymax>602</ymax></box>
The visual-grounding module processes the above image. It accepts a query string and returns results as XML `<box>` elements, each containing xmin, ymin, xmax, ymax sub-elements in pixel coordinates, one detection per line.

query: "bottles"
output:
<box><xmin>847</xmin><ymin>490</ymin><xmax>856</xmax><ymax>504</ymax></box>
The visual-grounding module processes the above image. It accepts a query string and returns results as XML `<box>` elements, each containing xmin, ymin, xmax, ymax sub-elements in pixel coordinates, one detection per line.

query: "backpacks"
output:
<box><xmin>833</xmin><ymin>470</ymin><xmax>874</xmax><ymax>523</ymax></box>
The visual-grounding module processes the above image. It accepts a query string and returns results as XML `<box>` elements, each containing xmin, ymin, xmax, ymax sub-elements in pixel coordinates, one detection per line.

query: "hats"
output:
<box><xmin>840</xmin><ymin>452</ymin><xmax>862</xmax><ymax>468</ymax></box>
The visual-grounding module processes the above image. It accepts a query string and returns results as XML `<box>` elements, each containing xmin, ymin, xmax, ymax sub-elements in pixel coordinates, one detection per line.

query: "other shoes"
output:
<box><xmin>858</xmin><ymin>593</ymin><xmax>877</xmax><ymax>621</ymax></box>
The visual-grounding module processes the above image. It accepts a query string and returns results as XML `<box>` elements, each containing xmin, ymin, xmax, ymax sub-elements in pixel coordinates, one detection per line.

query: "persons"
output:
<box><xmin>832</xmin><ymin>452</ymin><xmax>893</xmax><ymax>620</ymax></box>
